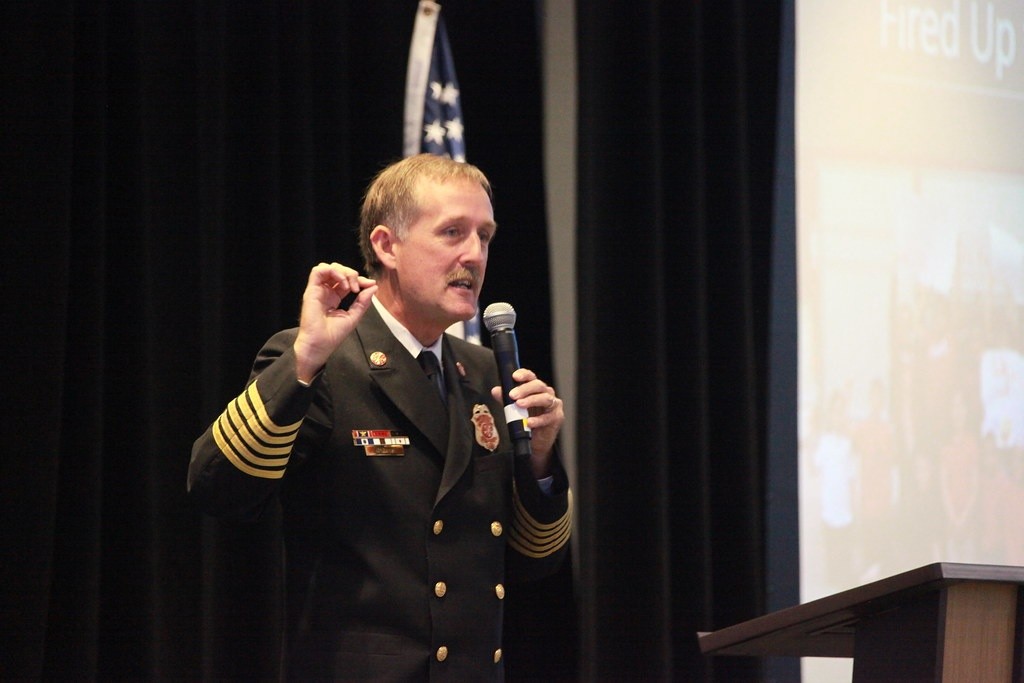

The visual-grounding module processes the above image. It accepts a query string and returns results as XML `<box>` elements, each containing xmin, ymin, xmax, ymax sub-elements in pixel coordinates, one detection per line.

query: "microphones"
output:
<box><xmin>483</xmin><ymin>302</ymin><xmax>533</xmax><ymax>459</ymax></box>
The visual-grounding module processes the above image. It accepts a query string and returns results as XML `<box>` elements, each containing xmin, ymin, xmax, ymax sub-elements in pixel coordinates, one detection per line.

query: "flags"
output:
<box><xmin>403</xmin><ymin>0</ymin><xmax>482</xmax><ymax>346</ymax></box>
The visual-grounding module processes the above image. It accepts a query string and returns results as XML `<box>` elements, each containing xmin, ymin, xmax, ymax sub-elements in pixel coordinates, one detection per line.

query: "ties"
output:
<box><xmin>417</xmin><ymin>350</ymin><xmax>448</xmax><ymax>414</ymax></box>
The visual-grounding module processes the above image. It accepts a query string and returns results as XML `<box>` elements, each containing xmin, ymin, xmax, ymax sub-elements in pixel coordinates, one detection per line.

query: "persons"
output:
<box><xmin>187</xmin><ymin>153</ymin><xmax>573</xmax><ymax>683</ymax></box>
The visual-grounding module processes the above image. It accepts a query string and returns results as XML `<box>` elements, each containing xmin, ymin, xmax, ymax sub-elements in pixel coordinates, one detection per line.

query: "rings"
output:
<box><xmin>548</xmin><ymin>399</ymin><xmax>555</xmax><ymax>409</ymax></box>
